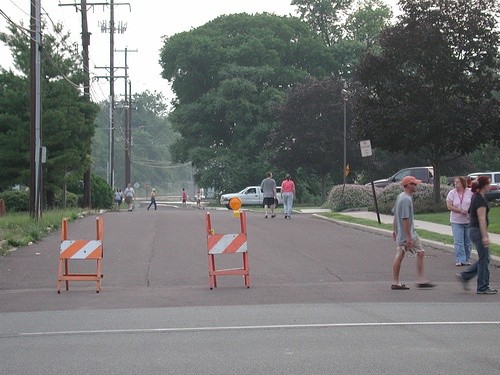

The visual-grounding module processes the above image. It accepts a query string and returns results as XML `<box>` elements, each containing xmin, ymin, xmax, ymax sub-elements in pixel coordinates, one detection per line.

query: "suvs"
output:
<box><xmin>220</xmin><ymin>185</ymin><xmax>284</xmax><ymax>210</ymax></box>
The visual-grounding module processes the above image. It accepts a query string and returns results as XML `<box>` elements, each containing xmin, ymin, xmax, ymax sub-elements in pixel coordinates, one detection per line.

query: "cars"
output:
<box><xmin>467</xmin><ymin>171</ymin><xmax>500</xmax><ymax>202</ymax></box>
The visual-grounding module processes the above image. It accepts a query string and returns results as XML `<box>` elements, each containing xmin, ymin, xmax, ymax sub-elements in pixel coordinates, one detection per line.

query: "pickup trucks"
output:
<box><xmin>365</xmin><ymin>166</ymin><xmax>473</xmax><ymax>189</ymax></box>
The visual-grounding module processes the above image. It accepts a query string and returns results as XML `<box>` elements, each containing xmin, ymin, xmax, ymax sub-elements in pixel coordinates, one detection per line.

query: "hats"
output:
<box><xmin>402</xmin><ymin>176</ymin><xmax>421</xmax><ymax>186</ymax></box>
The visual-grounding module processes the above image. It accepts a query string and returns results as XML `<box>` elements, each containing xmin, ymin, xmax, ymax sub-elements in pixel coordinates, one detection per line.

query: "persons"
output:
<box><xmin>124</xmin><ymin>184</ymin><xmax>136</xmax><ymax>211</ymax></box>
<box><xmin>148</xmin><ymin>189</ymin><xmax>156</xmax><ymax>211</ymax></box>
<box><xmin>197</xmin><ymin>192</ymin><xmax>201</xmax><ymax>206</ymax></box>
<box><xmin>391</xmin><ymin>176</ymin><xmax>435</xmax><ymax>289</ymax></box>
<box><xmin>181</xmin><ymin>188</ymin><xmax>187</xmax><ymax>207</ymax></box>
<box><xmin>260</xmin><ymin>172</ymin><xmax>277</xmax><ymax>218</ymax></box>
<box><xmin>281</xmin><ymin>174</ymin><xmax>295</xmax><ymax>219</ymax></box>
<box><xmin>446</xmin><ymin>176</ymin><xmax>474</xmax><ymax>266</ymax></box>
<box><xmin>114</xmin><ymin>188</ymin><xmax>123</xmax><ymax>211</ymax></box>
<box><xmin>456</xmin><ymin>176</ymin><xmax>497</xmax><ymax>294</ymax></box>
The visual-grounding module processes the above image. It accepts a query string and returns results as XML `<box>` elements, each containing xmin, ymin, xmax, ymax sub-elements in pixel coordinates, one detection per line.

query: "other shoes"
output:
<box><xmin>288</xmin><ymin>216</ymin><xmax>291</xmax><ymax>219</ymax></box>
<box><xmin>477</xmin><ymin>287</ymin><xmax>497</xmax><ymax>294</ymax></box>
<box><xmin>456</xmin><ymin>261</ymin><xmax>471</xmax><ymax>265</ymax></box>
<box><xmin>271</xmin><ymin>214</ymin><xmax>276</xmax><ymax>218</ymax></box>
<box><xmin>391</xmin><ymin>284</ymin><xmax>411</xmax><ymax>289</ymax></box>
<box><xmin>417</xmin><ymin>282</ymin><xmax>435</xmax><ymax>288</ymax></box>
<box><xmin>285</xmin><ymin>213</ymin><xmax>288</xmax><ymax>218</ymax></box>
<box><xmin>265</xmin><ymin>215</ymin><xmax>268</xmax><ymax>218</ymax></box>
<box><xmin>455</xmin><ymin>273</ymin><xmax>467</xmax><ymax>290</ymax></box>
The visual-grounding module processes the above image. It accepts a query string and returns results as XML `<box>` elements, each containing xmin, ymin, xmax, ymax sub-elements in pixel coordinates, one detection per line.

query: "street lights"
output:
<box><xmin>341</xmin><ymin>89</ymin><xmax>353</xmax><ymax>184</ymax></box>
